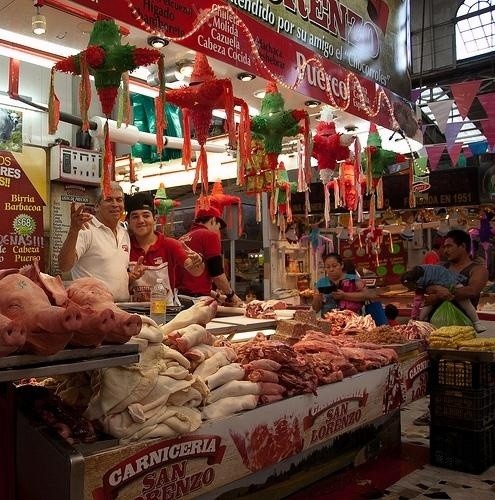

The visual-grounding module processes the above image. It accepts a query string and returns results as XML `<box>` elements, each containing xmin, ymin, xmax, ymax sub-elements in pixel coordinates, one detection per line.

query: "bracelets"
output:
<box><xmin>213</xmin><ymin>292</ymin><xmax>220</xmax><ymax>301</ymax></box>
<box><xmin>197</xmin><ymin>251</ymin><xmax>205</xmax><ymax>264</ymax></box>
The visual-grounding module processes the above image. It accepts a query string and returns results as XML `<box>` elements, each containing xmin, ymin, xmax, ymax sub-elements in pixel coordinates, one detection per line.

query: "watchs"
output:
<box><xmin>225</xmin><ymin>289</ymin><xmax>235</xmax><ymax>300</ymax></box>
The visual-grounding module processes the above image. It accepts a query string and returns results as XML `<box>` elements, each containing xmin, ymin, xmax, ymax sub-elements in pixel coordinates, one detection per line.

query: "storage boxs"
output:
<box><xmin>428</xmin><ymin>391</ymin><xmax>495</xmax><ymax>429</ymax></box>
<box><xmin>420</xmin><ymin>361</ymin><xmax>495</xmax><ymax>391</ymax></box>
<box><xmin>429</xmin><ymin>426</ymin><xmax>495</xmax><ymax>475</ymax></box>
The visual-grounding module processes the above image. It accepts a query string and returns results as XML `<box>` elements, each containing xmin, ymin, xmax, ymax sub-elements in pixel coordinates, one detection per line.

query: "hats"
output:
<box><xmin>194</xmin><ymin>205</ymin><xmax>227</xmax><ymax>230</ymax></box>
<box><xmin>123</xmin><ymin>191</ymin><xmax>158</xmax><ymax>217</ymax></box>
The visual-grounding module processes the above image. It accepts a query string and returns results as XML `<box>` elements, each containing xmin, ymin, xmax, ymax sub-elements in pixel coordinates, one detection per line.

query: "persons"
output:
<box><xmin>57</xmin><ymin>180</ymin><xmax>131</xmax><ymax>303</ymax></box>
<box><xmin>400</xmin><ymin>263</ymin><xmax>487</xmax><ymax>334</ymax></box>
<box><xmin>383</xmin><ymin>304</ymin><xmax>400</xmax><ymax>327</ymax></box>
<box><xmin>311</xmin><ymin>251</ymin><xmax>367</xmax><ymax>309</ymax></box>
<box><xmin>177</xmin><ymin>208</ymin><xmax>242</xmax><ymax>307</ymax></box>
<box><xmin>124</xmin><ymin>192</ymin><xmax>205</xmax><ymax>306</ymax></box>
<box><xmin>341</xmin><ymin>259</ymin><xmax>353</xmax><ymax>280</ymax></box>
<box><xmin>412</xmin><ymin>228</ymin><xmax>490</xmax><ymax>427</ymax></box>
<box><xmin>244</xmin><ymin>285</ymin><xmax>262</xmax><ymax>303</ymax></box>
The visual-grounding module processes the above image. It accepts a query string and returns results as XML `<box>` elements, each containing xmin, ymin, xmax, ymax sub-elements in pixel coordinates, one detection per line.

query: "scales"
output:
<box><xmin>360</xmin><ymin>276</ymin><xmax>387</xmax><ymax>289</ymax></box>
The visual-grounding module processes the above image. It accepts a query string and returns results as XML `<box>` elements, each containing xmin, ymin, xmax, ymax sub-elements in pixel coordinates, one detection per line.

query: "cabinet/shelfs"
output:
<box><xmin>0</xmin><ymin>303</ymin><xmax>431</xmax><ymax>500</ymax></box>
<box><xmin>270</xmin><ymin>240</ymin><xmax>311</xmax><ymax>303</ymax></box>
<box><xmin>300</xmin><ymin>282</ymin><xmax>495</xmax><ymax>337</ymax></box>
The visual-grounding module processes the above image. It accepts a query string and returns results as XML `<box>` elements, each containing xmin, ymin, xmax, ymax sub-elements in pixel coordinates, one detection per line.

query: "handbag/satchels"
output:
<box><xmin>472</xmin><ymin>322</ymin><xmax>487</xmax><ymax>333</ymax></box>
<box><xmin>364</xmin><ymin>297</ymin><xmax>388</xmax><ymax>326</ymax></box>
<box><xmin>414</xmin><ymin>410</ymin><xmax>431</xmax><ymax>426</ymax></box>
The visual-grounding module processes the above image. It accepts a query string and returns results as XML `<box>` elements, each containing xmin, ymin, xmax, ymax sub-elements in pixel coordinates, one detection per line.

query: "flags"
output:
<box><xmin>477</xmin><ymin>92</ymin><xmax>495</xmax><ymax>120</ymax></box>
<box><xmin>468</xmin><ymin>142</ymin><xmax>488</xmax><ymax>159</ymax></box>
<box><xmin>444</xmin><ymin>143</ymin><xmax>462</xmax><ymax>168</ymax></box>
<box><xmin>480</xmin><ymin>119</ymin><xmax>495</xmax><ymax>151</ymax></box>
<box><xmin>429</xmin><ymin>101</ymin><xmax>454</xmax><ymax>136</ymax></box>
<box><xmin>442</xmin><ymin>123</ymin><xmax>463</xmax><ymax>150</ymax></box>
<box><xmin>426</xmin><ymin>147</ymin><xmax>444</xmax><ymax>171</ymax></box>
<box><xmin>450</xmin><ymin>80</ymin><xmax>482</xmax><ymax>121</ymax></box>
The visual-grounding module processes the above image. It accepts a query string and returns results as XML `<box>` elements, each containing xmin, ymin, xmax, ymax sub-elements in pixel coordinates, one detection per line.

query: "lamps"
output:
<box><xmin>305</xmin><ymin>100</ymin><xmax>321</xmax><ymax>108</ymax></box>
<box><xmin>146</xmin><ymin>36</ymin><xmax>168</xmax><ymax>50</ymax></box>
<box><xmin>177</xmin><ymin>60</ymin><xmax>196</xmax><ymax>79</ymax></box>
<box><xmin>32</xmin><ymin>6</ymin><xmax>47</xmax><ymax>35</ymax></box>
<box><xmin>237</xmin><ymin>72</ymin><xmax>256</xmax><ymax>83</ymax></box>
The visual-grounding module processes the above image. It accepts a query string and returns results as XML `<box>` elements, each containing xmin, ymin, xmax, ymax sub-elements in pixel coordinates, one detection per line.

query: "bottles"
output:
<box><xmin>149</xmin><ymin>278</ymin><xmax>168</xmax><ymax>326</ymax></box>
